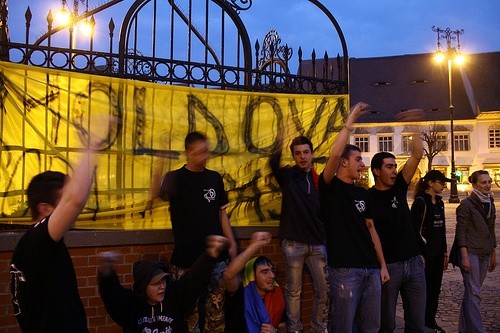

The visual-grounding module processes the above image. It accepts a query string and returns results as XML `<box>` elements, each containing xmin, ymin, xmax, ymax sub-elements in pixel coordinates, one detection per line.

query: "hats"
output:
<box><xmin>148</xmin><ymin>266</ymin><xmax>170</xmax><ymax>285</ymax></box>
<box><xmin>423</xmin><ymin>170</ymin><xmax>452</xmax><ymax>182</ymax></box>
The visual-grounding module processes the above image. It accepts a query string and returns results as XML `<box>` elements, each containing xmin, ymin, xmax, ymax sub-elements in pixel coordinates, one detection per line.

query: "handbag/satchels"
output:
<box><xmin>447</xmin><ymin>236</ymin><xmax>459</xmax><ymax>267</ymax></box>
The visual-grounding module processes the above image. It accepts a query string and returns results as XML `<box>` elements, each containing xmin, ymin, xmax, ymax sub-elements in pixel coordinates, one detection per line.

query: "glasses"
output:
<box><xmin>435</xmin><ymin>182</ymin><xmax>444</xmax><ymax>185</ymax></box>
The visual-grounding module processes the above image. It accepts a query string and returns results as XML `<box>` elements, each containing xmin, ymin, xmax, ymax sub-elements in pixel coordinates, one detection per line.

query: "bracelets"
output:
<box><xmin>343</xmin><ymin>124</ymin><xmax>354</xmax><ymax>132</ymax></box>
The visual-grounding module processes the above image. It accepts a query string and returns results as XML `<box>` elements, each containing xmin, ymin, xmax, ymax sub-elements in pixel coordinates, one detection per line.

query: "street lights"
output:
<box><xmin>54</xmin><ymin>0</ymin><xmax>94</xmax><ymax>72</ymax></box>
<box><xmin>432</xmin><ymin>26</ymin><xmax>466</xmax><ymax>202</ymax></box>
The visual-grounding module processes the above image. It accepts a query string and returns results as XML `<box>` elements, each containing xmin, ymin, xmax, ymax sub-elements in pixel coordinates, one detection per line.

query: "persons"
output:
<box><xmin>226</xmin><ymin>230</ymin><xmax>288</xmax><ymax>333</ymax></box>
<box><xmin>8</xmin><ymin>115</ymin><xmax>102</xmax><ymax>333</ymax></box>
<box><xmin>97</xmin><ymin>234</ymin><xmax>228</xmax><ymax>333</ymax></box>
<box><xmin>407</xmin><ymin>169</ymin><xmax>456</xmax><ymax>333</ymax></box>
<box><xmin>153</xmin><ymin>131</ymin><xmax>238</xmax><ymax>333</ymax></box>
<box><xmin>318</xmin><ymin>100</ymin><xmax>391</xmax><ymax>333</ymax></box>
<box><xmin>364</xmin><ymin>108</ymin><xmax>427</xmax><ymax>333</ymax></box>
<box><xmin>455</xmin><ymin>169</ymin><xmax>497</xmax><ymax>333</ymax></box>
<box><xmin>269</xmin><ymin>117</ymin><xmax>330</xmax><ymax>333</ymax></box>
